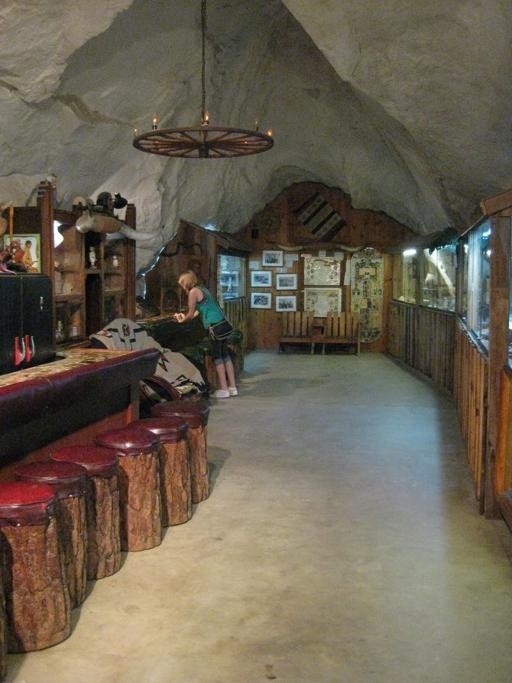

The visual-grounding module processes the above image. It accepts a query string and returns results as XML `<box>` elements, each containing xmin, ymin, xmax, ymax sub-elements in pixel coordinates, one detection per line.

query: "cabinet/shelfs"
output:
<box><xmin>0</xmin><ymin>273</ymin><xmax>54</xmax><ymax>376</ymax></box>
<box><xmin>2</xmin><ymin>177</ymin><xmax>136</xmax><ymax>352</ymax></box>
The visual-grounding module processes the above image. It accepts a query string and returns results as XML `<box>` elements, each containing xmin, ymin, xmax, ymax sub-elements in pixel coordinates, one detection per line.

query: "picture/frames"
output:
<box><xmin>248</xmin><ymin>249</ymin><xmax>342</xmax><ymax>318</ymax></box>
<box><xmin>3</xmin><ymin>232</ymin><xmax>42</xmax><ymax>275</ymax></box>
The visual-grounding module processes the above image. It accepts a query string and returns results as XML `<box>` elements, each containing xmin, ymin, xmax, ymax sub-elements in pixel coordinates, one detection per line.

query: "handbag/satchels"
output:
<box><xmin>208</xmin><ymin>319</ymin><xmax>233</xmax><ymax>342</ymax></box>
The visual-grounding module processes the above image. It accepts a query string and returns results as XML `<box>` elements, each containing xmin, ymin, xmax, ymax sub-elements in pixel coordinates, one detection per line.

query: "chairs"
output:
<box><xmin>312</xmin><ymin>311</ymin><xmax>360</xmax><ymax>357</ymax></box>
<box><xmin>278</xmin><ymin>310</ymin><xmax>316</xmax><ymax>354</ymax></box>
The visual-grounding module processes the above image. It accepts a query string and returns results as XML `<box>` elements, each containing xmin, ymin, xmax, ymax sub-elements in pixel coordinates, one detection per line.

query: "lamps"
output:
<box><xmin>132</xmin><ymin>1</ymin><xmax>274</xmax><ymax>160</ymax></box>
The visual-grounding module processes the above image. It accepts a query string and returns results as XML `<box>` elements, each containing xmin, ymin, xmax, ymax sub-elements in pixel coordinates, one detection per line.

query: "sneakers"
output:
<box><xmin>228</xmin><ymin>387</ymin><xmax>238</xmax><ymax>396</ymax></box>
<box><xmin>209</xmin><ymin>389</ymin><xmax>229</xmax><ymax>397</ymax></box>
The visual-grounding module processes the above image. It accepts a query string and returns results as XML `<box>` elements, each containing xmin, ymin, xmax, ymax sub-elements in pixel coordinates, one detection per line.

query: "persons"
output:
<box><xmin>0</xmin><ymin>236</ymin><xmax>37</xmax><ymax>275</ymax></box>
<box><xmin>174</xmin><ymin>267</ymin><xmax>238</xmax><ymax>400</ymax></box>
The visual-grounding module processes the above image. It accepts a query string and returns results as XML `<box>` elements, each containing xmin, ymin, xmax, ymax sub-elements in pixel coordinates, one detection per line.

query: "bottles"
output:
<box><xmin>112</xmin><ymin>255</ymin><xmax>120</xmax><ymax>269</ymax></box>
<box><xmin>56</xmin><ymin>320</ymin><xmax>65</xmax><ymax>341</ymax></box>
